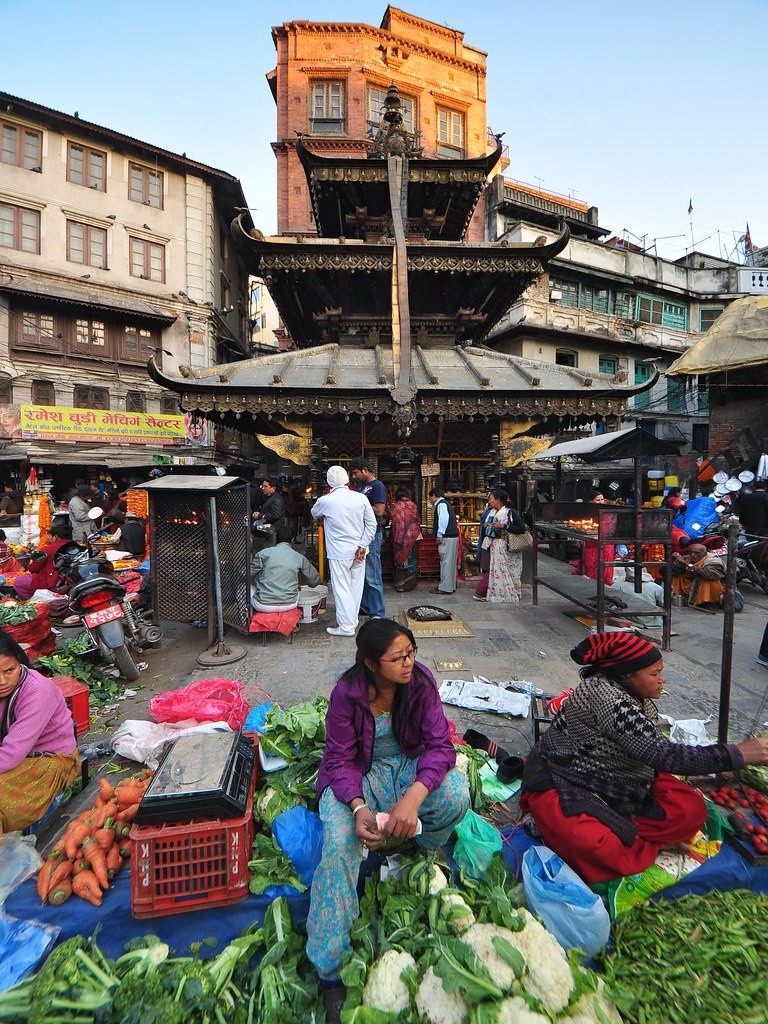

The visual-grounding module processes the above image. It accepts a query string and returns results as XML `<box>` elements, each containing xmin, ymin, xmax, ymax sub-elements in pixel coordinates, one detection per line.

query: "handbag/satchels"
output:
<box><xmin>507</xmin><ymin>512</ymin><xmax>533</xmax><ymax>552</ymax></box>
<box><xmin>521</xmin><ymin>844</ymin><xmax>611</xmax><ymax>962</ymax></box>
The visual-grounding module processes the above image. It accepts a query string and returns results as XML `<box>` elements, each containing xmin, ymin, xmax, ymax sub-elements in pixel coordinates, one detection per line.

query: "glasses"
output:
<box><xmin>380</xmin><ymin>646</ymin><xmax>419</xmax><ymax>664</ymax></box>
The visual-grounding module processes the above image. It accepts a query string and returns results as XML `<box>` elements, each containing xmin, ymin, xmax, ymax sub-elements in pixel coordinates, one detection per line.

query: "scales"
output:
<box><xmin>133</xmin><ymin>729</ymin><xmax>257</xmax><ymax>825</ymax></box>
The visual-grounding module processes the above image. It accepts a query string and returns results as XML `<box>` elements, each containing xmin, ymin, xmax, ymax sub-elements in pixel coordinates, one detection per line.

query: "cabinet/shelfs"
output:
<box><xmin>648</xmin><ymin>478</ymin><xmax>667</xmax><ymax>507</ymax></box>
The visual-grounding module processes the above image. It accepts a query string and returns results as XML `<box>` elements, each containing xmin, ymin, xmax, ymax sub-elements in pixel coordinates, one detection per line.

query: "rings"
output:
<box><xmin>363</xmin><ymin>839</ymin><xmax>367</xmax><ymax>849</ymax></box>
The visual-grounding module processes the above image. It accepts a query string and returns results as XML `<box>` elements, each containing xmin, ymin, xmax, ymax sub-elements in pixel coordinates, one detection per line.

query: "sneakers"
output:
<box><xmin>326</xmin><ymin>627</ymin><xmax>355</xmax><ymax>637</ymax></box>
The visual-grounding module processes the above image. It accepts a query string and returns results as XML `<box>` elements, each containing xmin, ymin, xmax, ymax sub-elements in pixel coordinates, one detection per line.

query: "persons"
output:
<box><xmin>0</xmin><ymin>481</ymin><xmax>24</xmax><ymax>516</ymax></box>
<box><xmin>661</xmin><ymin>487</ymin><xmax>682</xmax><ymax>506</ymax></box>
<box><xmin>0</xmin><ymin>529</ymin><xmax>14</xmax><ymax>566</ymax></box>
<box><xmin>14</xmin><ymin>527</ymin><xmax>72</xmax><ymax>600</ymax></box>
<box><xmin>66</xmin><ymin>479</ymin><xmax>146</xmax><ymax>555</ymax></box>
<box><xmin>252</xmin><ymin>476</ymin><xmax>304</xmax><ymax>544</ymax></box>
<box><xmin>236</xmin><ymin>526</ymin><xmax>320</xmax><ymax>629</ymax></box>
<box><xmin>518</xmin><ymin>631</ymin><xmax>768</xmax><ymax>885</ymax></box>
<box><xmin>472</xmin><ymin>489</ymin><xmax>525</xmax><ymax>602</ymax></box>
<box><xmin>429</xmin><ymin>487</ymin><xmax>459</xmax><ymax>595</ymax></box>
<box><xmin>756</xmin><ymin>621</ymin><xmax>768</xmax><ymax>670</ymax></box>
<box><xmin>311</xmin><ymin>465</ymin><xmax>378</xmax><ymax>637</ymax></box>
<box><xmin>304</xmin><ymin>619</ymin><xmax>472</xmax><ymax>1024</ymax></box>
<box><xmin>0</xmin><ymin>630</ymin><xmax>80</xmax><ymax>836</ymax></box>
<box><xmin>390</xmin><ymin>488</ymin><xmax>424</xmax><ymax>592</ymax></box>
<box><xmin>348</xmin><ymin>457</ymin><xmax>385</xmax><ymax>620</ymax></box>
<box><xmin>658</xmin><ymin>543</ymin><xmax>744</xmax><ymax>612</ymax></box>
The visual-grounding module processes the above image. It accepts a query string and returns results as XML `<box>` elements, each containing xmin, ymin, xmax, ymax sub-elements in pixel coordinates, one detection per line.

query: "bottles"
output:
<box><xmin>677</xmin><ymin>594</ymin><xmax>682</xmax><ymax>606</ymax></box>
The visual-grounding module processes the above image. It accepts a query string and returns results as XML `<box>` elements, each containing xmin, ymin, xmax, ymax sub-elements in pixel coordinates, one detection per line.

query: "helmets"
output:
<box><xmin>669</xmin><ymin>496</ymin><xmax>688</xmax><ymax>516</ymax></box>
<box><xmin>251</xmin><ymin>518</ymin><xmax>271</xmax><ymax>539</ymax></box>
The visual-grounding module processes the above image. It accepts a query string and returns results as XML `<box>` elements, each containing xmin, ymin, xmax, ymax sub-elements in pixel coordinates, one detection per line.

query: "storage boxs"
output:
<box><xmin>19</xmin><ymin>537</ymin><xmax>40</xmax><ymax>547</ymax></box>
<box><xmin>651</xmin><ymin>495</ymin><xmax>665</xmax><ymax>507</ymax></box>
<box><xmin>128</xmin><ymin>732</ymin><xmax>260</xmax><ymax>920</ymax></box>
<box><xmin>648</xmin><ymin>470</ymin><xmax>666</xmax><ymax>478</ymax></box>
<box><xmin>32</xmin><ymin>495</ymin><xmax>43</xmax><ymax>505</ymax></box>
<box><xmin>648</xmin><ymin>479</ymin><xmax>664</xmax><ymax>490</ymax></box>
<box><xmin>19</xmin><ymin>527</ymin><xmax>40</xmax><ymax>538</ymax></box>
<box><xmin>664</xmin><ymin>476</ymin><xmax>679</xmax><ymax>487</ymax></box>
<box><xmin>20</xmin><ymin>515</ymin><xmax>38</xmax><ymax>529</ymax></box>
<box><xmin>23</xmin><ymin>504</ymin><xmax>39</xmax><ymax>515</ymax></box>
<box><xmin>662</xmin><ymin>486</ymin><xmax>673</xmax><ymax>497</ymax></box>
<box><xmin>22</xmin><ymin>495</ymin><xmax>32</xmax><ymax>505</ymax></box>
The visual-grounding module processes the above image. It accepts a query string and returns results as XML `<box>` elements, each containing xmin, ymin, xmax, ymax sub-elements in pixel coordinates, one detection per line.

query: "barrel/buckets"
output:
<box><xmin>295</xmin><ymin>590</ymin><xmax>320</xmax><ymax>624</ymax></box>
<box><xmin>300</xmin><ymin>584</ymin><xmax>328</xmax><ymax>614</ymax></box>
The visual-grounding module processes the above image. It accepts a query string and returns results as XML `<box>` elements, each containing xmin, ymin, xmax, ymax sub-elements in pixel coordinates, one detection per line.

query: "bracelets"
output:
<box><xmin>353</xmin><ymin>804</ymin><xmax>367</xmax><ymax>818</ymax></box>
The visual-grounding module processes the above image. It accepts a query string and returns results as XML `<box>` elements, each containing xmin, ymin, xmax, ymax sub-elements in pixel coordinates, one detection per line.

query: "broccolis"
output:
<box><xmin>256</xmin><ymin>784</ymin><xmax>309</xmax><ymax>823</ymax></box>
<box><xmin>5</xmin><ymin>600</ymin><xmax>17</xmax><ymax>607</ymax></box>
<box><xmin>0</xmin><ymin>936</ymin><xmax>299</xmax><ymax>1024</ymax></box>
<box><xmin>361</xmin><ymin>865</ymin><xmax>628</xmax><ymax>1024</ymax></box>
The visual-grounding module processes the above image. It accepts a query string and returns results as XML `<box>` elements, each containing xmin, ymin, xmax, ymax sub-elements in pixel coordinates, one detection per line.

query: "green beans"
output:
<box><xmin>589</xmin><ymin>887</ymin><xmax>768</xmax><ymax>1024</ymax></box>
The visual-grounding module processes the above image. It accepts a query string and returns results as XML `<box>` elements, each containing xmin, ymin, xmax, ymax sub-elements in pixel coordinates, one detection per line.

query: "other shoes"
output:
<box><xmin>756</xmin><ymin>654</ymin><xmax>768</xmax><ymax>666</ymax></box>
<box><xmin>434</xmin><ymin>586</ymin><xmax>455</xmax><ymax>592</ymax></box>
<box><xmin>473</xmin><ymin>593</ymin><xmax>484</xmax><ymax>601</ymax></box>
<box><xmin>430</xmin><ymin>589</ymin><xmax>450</xmax><ymax>595</ymax></box>
<box><xmin>323</xmin><ymin>986</ymin><xmax>345</xmax><ymax>1024</ymax></box>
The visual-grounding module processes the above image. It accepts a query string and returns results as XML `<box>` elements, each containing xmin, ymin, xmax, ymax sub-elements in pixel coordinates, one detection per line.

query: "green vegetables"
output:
<box><xmin>737</xmin><ymin>730</ymin><xmax>768</xmax><ymax>794</ymax></box>
<box><xmin>0</xmin><ymin>602</ymin><xmax>37</xmax><ymax>628</ymax></box>
<box><xmin>37</xmin><ymin>630</ymin><xmax>144</xmax><ymax>707</ymax></box>
<box><xmin>246</xmin><ymin>833</ymin><xmax>327</xmax><ymax>1024</ymax></box>
<box><xmin>260</xmin><ymin>696</ymin><xmax>330</xmax><ymax>799</ymax></box>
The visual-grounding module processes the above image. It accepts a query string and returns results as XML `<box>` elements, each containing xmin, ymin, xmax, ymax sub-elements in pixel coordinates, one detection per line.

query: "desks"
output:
<box><xmin>380</xmin><ymin>532</ymin><xmax>442</xmax><ymax>579</ymax></box>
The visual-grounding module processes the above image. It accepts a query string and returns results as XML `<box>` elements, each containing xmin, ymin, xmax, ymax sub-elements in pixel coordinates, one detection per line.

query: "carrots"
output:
<box><xmin>34</xmin><ymin>767</ymin><xmax>156</xmax><ymax>906</ymax></box>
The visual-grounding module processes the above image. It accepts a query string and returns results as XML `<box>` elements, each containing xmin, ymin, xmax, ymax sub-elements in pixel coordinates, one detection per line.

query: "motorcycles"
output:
<box><xmin>692</xmin><ymin>504</ymin><xmax>768</xmax><ymax>593</ymax></box>
<box><xmin>30</xmin><ymin>507</ymin><xmax>164</xmax><ymax>682</ymax></box>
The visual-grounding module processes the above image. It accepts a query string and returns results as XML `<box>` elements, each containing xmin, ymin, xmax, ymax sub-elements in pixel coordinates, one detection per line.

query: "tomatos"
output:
<box><xmin>710</xmin><ymin>784</ymin><xmax>768</xmax><ymax>857</ymax></box>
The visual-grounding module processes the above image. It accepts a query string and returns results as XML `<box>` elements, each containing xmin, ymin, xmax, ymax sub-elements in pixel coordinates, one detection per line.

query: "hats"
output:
<box><xmin>570</xmin><ymin>632</ymin><xmax>661</xmax><ymax>679</ymax></box>
<box><xmin>326</xmin><ymin>465</ymin><xmax>348</xmax><ymax>487</ymax></box>
<box><xmin>124</xmin><ymin>514</ymin><xmax>138</xmax><ymax>518</ymax></box>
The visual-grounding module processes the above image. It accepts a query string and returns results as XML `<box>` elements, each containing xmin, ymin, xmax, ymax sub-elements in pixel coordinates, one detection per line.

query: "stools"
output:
<box><xmin>79</xmin><ymin>754</ymin><xmax>90</xmax><ymax>791</ymax></box>
<box><xmin>51</xmin><ymin>674</ymin><xmax>91</xmax><ymax>736</ymax></box>
<box><xmin>249</xmin><ymin>608</ymin><xmax>302</xmax><ymax>647</ymax></box>
<box><xmin>531</xmin><ymin>692</ymin><xmax>559</xmax><ymax>744</ymax></box>
